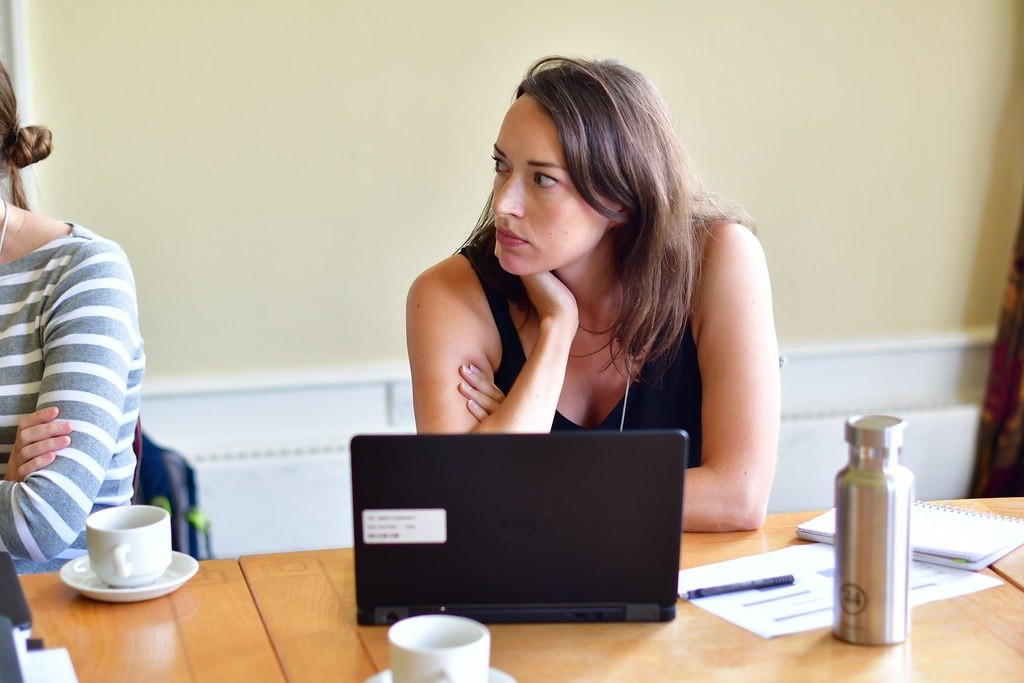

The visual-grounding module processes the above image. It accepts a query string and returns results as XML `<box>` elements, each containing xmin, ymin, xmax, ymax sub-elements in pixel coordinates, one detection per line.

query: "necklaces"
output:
<box><xmin>0</xmin><ymin>197</ymin><xmax>9</xmax><ymax>254</ymax></box>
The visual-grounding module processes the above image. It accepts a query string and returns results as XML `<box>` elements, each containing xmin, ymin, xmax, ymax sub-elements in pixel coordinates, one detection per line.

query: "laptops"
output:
<box><xmin>348</xmin><ymin>429</ymin><xmax>688</xmax><ymax>626</ymax></box>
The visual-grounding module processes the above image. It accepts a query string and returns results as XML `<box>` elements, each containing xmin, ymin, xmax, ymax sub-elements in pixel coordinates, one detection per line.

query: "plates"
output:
<box><xmin>364</xmin><ymin>666</ymin><xmax>516</xmax><ymax>683</ymax></box>
<box><xmin>59</xmin><ymin>551</ymin><xmax>199</xmax><ymax>602</ymax></box>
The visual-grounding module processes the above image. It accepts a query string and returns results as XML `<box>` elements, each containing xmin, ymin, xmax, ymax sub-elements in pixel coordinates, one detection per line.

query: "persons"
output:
<box><xmin>0</xmin><ymin>63</ymin><xmax>145</xmax><ymax>575</ymax></box>
<box><xmin>405</xmin><ymin>59</ymin><xmax>778</xmax><ymax>531</ymax></box>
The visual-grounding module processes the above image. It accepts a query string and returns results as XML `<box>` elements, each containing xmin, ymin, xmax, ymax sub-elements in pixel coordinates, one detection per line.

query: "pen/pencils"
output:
<box><xmin>678</xmin><ymin>573</ymin><xmax>795</xmax><ymax>601</ymax></box>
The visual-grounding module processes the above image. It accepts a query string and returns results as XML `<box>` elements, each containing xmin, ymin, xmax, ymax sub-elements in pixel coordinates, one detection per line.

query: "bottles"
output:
<box><xmin>831</xmin><ymin>414</ymin><xmax>915</xmax><ymax>645</ymax></box>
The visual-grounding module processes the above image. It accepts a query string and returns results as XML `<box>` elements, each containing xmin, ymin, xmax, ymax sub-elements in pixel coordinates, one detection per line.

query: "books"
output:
<box><xmin>794</xmin><ymin>500</ymin><xmax>1024</xmax><ymax>572</ymax></box>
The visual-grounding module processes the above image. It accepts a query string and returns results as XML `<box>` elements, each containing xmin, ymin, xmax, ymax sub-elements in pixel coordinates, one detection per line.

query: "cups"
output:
<box><xmin>85</xmin><ymin>505</ymin><xmax>172</xmax><ymax>587</ymax></box>
<box><xmin>387</xmin><ymin>614</ymin><xmax>491</xmax><ymax>683</ymax></box>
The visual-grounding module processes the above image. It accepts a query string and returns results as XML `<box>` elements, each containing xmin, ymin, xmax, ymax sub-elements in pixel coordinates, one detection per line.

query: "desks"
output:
<box><xmin>238</xmin><ymin>497</ymin><xmax>1024</xmax><ymax>683</ymax></box>
<box><xmin>19</xmin><ymin>557</ymin><xmax>287</xmax><ymax>683</ymax></box>
